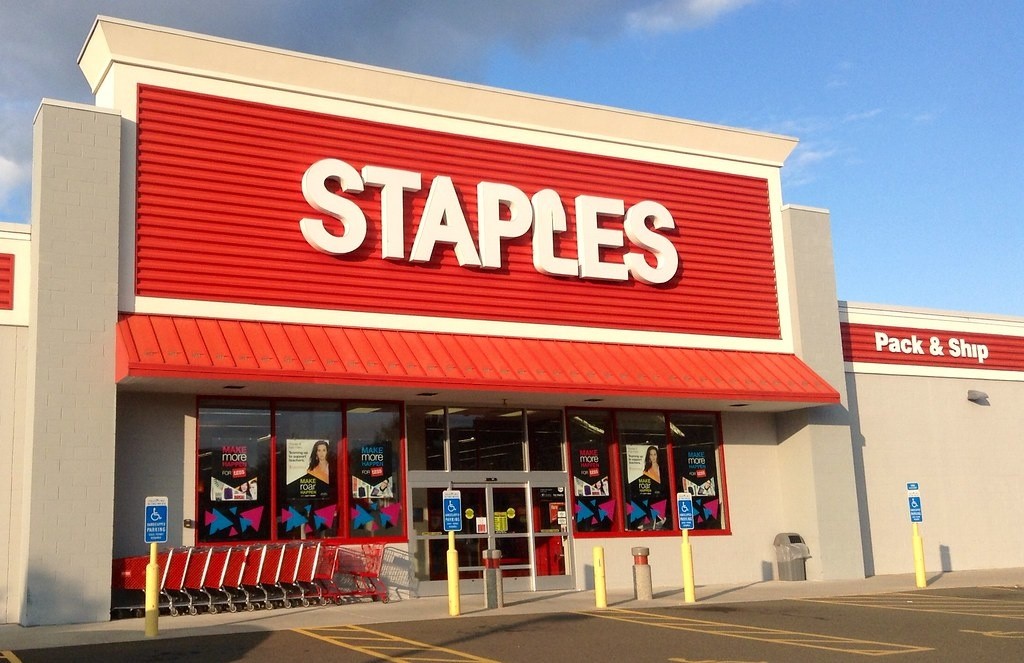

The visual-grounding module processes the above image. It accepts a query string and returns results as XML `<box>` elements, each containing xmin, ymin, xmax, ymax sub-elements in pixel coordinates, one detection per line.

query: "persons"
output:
<box><xmin>306</xmin><ymin>441</ymin><xmax>330</xmax><ymax>484</ymax></box>
<box><xmin>642</xmin><ymin>446</ymin><xmax>661</xmax><ymax>484</ymax></box>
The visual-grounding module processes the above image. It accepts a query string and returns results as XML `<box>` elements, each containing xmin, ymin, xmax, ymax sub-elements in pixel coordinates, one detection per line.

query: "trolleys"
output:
<box><xmin>109</xmin><ymin>539</ymin><xmax>390</xmax><ymax>618</ymax></box>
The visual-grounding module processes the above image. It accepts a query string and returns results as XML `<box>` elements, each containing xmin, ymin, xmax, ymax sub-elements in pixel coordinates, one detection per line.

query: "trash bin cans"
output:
<box><xmin>773</xmin><ymin>532</ymin><xmax>807</xmax><ymax>582</ymax></box>
